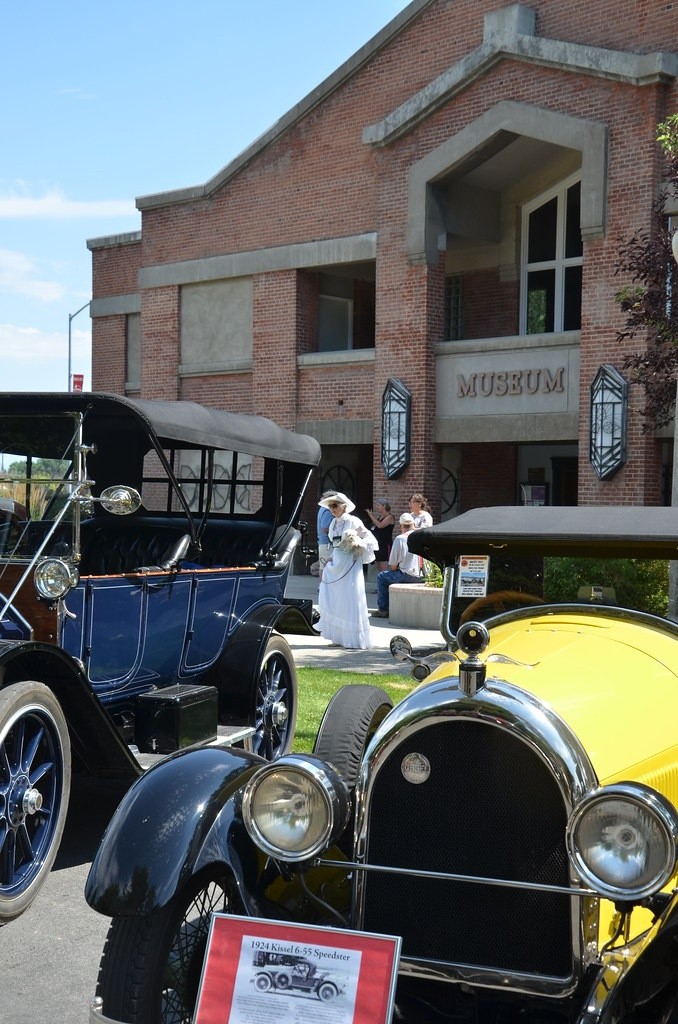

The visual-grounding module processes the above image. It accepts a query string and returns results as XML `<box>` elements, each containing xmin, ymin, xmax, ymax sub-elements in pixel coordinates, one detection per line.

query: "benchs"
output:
<box><xmin>61</xmin><ymin>514</ymin><xmax>303</xmax><ymax>577</ymax></box>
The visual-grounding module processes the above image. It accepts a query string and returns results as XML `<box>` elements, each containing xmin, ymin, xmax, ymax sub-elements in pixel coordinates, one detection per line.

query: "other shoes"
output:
<box><xmin>328</xmin><ymin>642</ymin><xmax>342</xmax><ymax>647</ymax></box>
<box><xmin>371</xmin><ymin>609</ymin><xmax>389</xmax><ymax>617</ymax></box>
<box><xmin>317</xmin><ymin>589</ymin><xmax>319</xmax><ymax>594</ymax></box>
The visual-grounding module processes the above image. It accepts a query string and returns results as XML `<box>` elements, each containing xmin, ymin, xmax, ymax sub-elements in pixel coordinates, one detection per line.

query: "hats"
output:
<box><xmin>399</xmin><ymin>512</ymin><xmax>414</xmax><ymax>525</ymax></box>
<box><xmin>378</xmin><ymin>499</ymin><xmax>390</xmax><ymax>511</ymax></box>
<box><xmin>318</xmin><ymin>493</ymin><xmax>356</xmax><ymax>513</ymax></box>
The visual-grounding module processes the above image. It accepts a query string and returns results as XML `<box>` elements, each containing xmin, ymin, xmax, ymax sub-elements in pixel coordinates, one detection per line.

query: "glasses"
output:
<box><xmin>327</xmin><ymin>503</ymin><xmax>343</xmax><ymax>509</ymax></box>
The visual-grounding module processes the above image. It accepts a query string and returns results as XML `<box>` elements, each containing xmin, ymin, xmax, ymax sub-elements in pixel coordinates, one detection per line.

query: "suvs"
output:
<box><xmin>83</xmin><ymin>503</ymin><xmax>678</xmax><ymax>1024</ymax></box>
<box><xmin>246</xmin><ymin>949</ymin><xmax>345</xmax><ymax>1002</ymax></box>
<box><xmin>1</xmin><ymin>388</ymin><xmax>327</xmax><ymax>920</ymax></box>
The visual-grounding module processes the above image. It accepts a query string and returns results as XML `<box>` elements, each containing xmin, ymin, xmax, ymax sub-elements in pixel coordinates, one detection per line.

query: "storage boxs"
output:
<box><xmin>134</xmin><ymin>685</ymin><xmax>218</xmax><ymax>754</ymax></box>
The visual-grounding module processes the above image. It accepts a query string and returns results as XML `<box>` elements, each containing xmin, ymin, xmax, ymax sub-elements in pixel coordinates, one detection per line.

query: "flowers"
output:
<box><xmin>338</xmin><ymin>529</ymin><xmax>362</xmax><ymax>552</ymax></box>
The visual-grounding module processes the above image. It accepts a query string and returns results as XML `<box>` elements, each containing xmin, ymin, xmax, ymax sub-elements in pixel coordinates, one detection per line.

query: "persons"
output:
<box><xmin>370</xmin><ymin>512</ymin><xmax>427</xmax><ymax>617</ymax></box>
<box><xmin>409</xmin><ymin>494</ymin><xmax>433</xmax><ymax>530</ymax></box>
<box><xmin>312</xmin><ymin>493</ymin><xmax>381</xmax><ymax>650</ymax></box>
<box><xmin>364</xmin><ymin>498</ymin><xmax>395</xmax><ymax>593</ymax></box>
<box><xmin>316</xmin><ymin>490</ymin><xmax>335</xmax><ymax>595</ymax></box>
